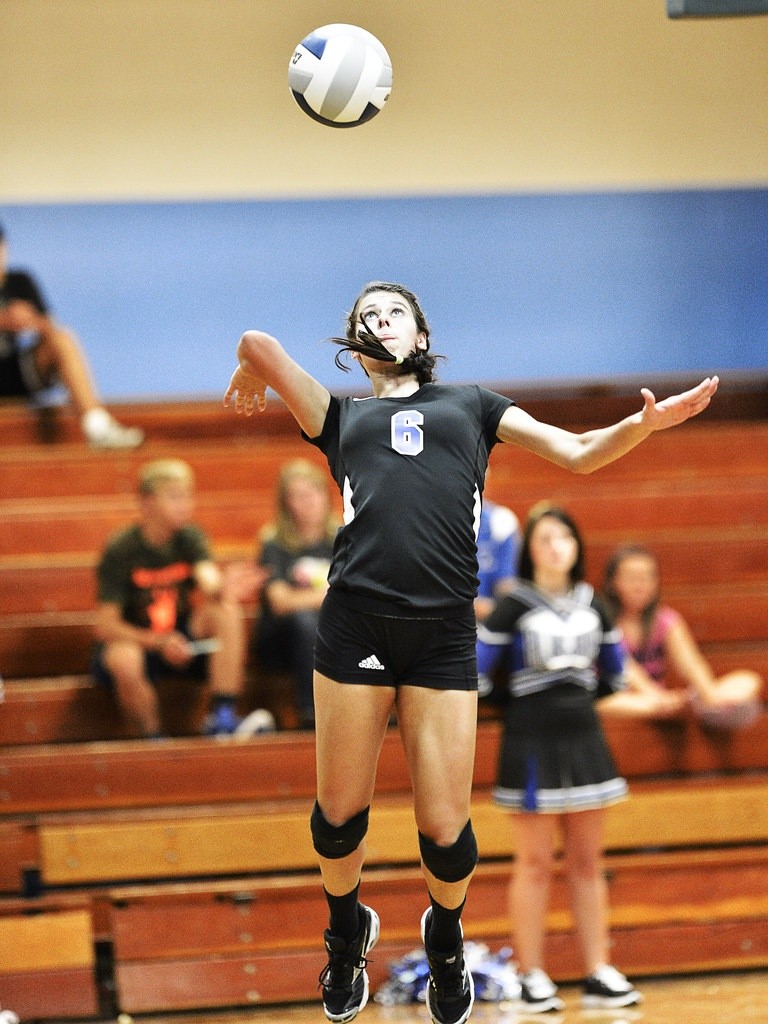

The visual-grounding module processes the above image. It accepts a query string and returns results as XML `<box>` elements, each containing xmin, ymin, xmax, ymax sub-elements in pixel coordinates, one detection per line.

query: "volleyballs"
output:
<box><xmin>286</xmin><ymin>22</ymin><xmax>397</xmax><ymax>131</ymax></box>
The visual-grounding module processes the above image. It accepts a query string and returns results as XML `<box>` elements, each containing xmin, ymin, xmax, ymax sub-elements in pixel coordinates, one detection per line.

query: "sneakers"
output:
<box><xmin>317</xmin><ymin>899</ymin><xmax>379</xmax><ymax>1024</ymax></box>
<box><xmin>518</xmin><ymin>968</ymin><xmax>565</xmax><ymax>1014</ymax></box>
<box><xmin>419</xmin><ymin>905</ymin><xmax>474</xmax><ymax>1024</ymax></box>
<box><xmin>581</xmin><ymin>965</ymin><xmax>643</xmax><ymax>1008</ymax></box>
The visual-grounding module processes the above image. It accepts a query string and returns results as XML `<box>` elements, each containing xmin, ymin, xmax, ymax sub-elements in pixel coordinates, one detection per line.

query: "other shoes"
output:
<box><xmin>82</xmin><ymin>405</ymin><xmax>144</xmax><ymax>451</ymax></box>
<box><xmin>211</xmin><ymin>706</ymin><xmax>278</xmax><ymax>736</ymax></box>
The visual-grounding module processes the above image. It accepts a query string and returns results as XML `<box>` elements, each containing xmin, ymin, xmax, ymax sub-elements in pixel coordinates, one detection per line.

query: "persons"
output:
<box><xmin>92</xmin><ymin>457</ymin><xmax>279</xmax><ymax>736</ymax></box>
<box><xmin>220</xmin><ymin>281</ymin><xmax>763</xmax><ymax>1024</ymax></box>
<box><xmin>0</xmin><ymin>222</ymin><xmax>146</xmax><ymax>451</ymax></box>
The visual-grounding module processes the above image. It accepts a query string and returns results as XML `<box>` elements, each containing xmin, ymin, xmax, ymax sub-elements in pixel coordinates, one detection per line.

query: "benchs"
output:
<box><xmin>1</xmin><ymin>375</ymin><xmax>768</xmax><ymax>1023</ymax></box>
